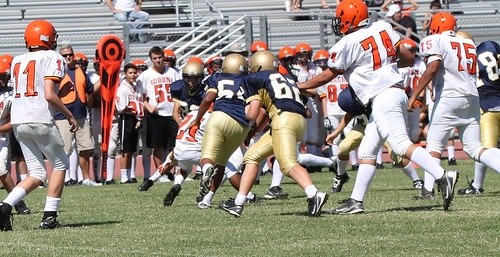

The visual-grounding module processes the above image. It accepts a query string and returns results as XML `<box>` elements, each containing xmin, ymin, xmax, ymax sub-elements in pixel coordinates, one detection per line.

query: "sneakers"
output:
<box><xmin>0</xmin><ymin>174</ymin><xmax>500</xmax><ymax>231</ymax></box>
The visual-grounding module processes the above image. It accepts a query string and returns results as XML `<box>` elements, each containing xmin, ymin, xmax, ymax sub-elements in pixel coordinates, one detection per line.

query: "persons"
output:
<box><xmin>291</xmin><ymin>0</ymin><xmax>340</xmax><ymax>10</ymax></box>
<box><xmin>423</xmin><ymin>0</ymin><xmax>442</xmax><ymax>35</ymax></box>
<box><xmin>381</xmin><ymin>0</ymin><xmax>420</xmax><ymax>42</ymax></box>
<box><xmin>295</xmin><ymin>0</ymin><xmax>459</xmax><ymax>214</ymax></box>
<box><xmin>407</xmin><ymin>11</ymin><xmax>500</xmax><ymax>200</ymax></box>
<box><xmin>0</xmin><ymin>30</ymin><xmax>500</xmax><ymax>214</ymax></box>
<box><xmin>187</xmin><ymin>53</ymin><xmax>252</xmax><ymax>209</ymax></box>
<box><xmin>0</xmin><ymin>20</ymin><xmax>78</xmax><ymax>229</ymax></box>
<box><xmin>106</xmin><ymin>0</ymin><xmax>150</xmax><ymax>41</ymax></box>
<box><xmin>219</xmin><ymin>49</ymin><xmax>328</xmax><ymax>218</ymax></box>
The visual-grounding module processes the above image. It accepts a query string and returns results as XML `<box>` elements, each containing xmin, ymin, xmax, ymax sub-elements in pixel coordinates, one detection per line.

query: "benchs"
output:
<box><xmin>0</xmin><ymin>0</ymin><xmax>500</xmax><ymax>78</ymax></box>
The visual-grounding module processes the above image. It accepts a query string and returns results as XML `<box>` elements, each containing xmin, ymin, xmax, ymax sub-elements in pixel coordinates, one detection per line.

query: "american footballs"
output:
<box><xmin>402</xmin><ymin>39</ymin><xmax>417</xmax><ymax>54</ymax></box>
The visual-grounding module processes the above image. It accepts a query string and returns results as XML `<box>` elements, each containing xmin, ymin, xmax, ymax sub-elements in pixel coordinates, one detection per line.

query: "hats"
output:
<box><xmin>385</xmin><ymin>4</ymin><xmax>401</xmax><ymax>17</ymax></box>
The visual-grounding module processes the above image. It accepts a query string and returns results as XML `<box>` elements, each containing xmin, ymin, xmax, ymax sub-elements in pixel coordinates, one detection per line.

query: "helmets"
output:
<box><xmin>182</xmin><ymin>62</ymin><xmax>203</xmax><ymax>78</ymax></box>
<box><xmin>249</xmin><ymin>51</ymin><xmax>277</xmax><ymax>72</ymax></box>
<box><xmin>130</xmin><ymin>60</ymin><xmax>147</xmax><ymax>70</ymax></box>
<box><xmin>398</xmin><ymin>38</ymin><xmax>416</xmax><ymax>56</ymax></box>
<box><xmin>250</xmin><ymin>42</ymin><xmax>268</xmax><ymax>52</ymax></box>
<box><xmin>456</xmin><ymin>30</ymin><xmax>474</xmax><ymax>43</ymax></box>
<box><xmin>313</xmin><ymin>50</ymin><xmax>329</xmax><ymax>63</ymax></box>
<box><xmin>163</xmin><ymin>50</ymin><xmax>176</xmax><ymax>65</ymax></box>
<box><xmin>429</xmin><ymin>12</ymin><xmax>458</xmax><ymax>34</ymax></box>
<box><xmin>279</xmin><ymin>47</ymin><xmax>295</xmax><ymax>59</ymax></box>
<box><xmin>188</xmin><ymin>57</ymin><xmax>202</xmax><ymax>65</ymax></box>
<box><xmin>222</xmin><ymin>54</ymin><xmax>248</xmax><ymax>75</ymax></box>
<box><xmin>332</xmin><ymin>0</ymin><xmax>369</xmax><ymax>36</ymax></box>
<box><xmin>24</xmin><ymin>20</ymin><xmax>57</xmax><ymax>50</ymax></box>
<box><xmin>73</xmin><ymin>53</ymin><xmax>88</xmax><ymax>71</ymax></box>
<box><xmin>294</xmin><ymin>44</ymin><xmax>313</xmax><ymax>61</ymax></box>
<box><xmin>0</xmin><ymin>53</ymin><xmax>13</xmax><ymax>73</ymax></box>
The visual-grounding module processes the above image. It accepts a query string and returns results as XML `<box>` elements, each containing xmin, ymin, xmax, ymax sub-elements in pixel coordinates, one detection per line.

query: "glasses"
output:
<box><xmin>62</xmin><ymin>54</ymin><xmax>73</xmax><ymax>58</ymax></box>
